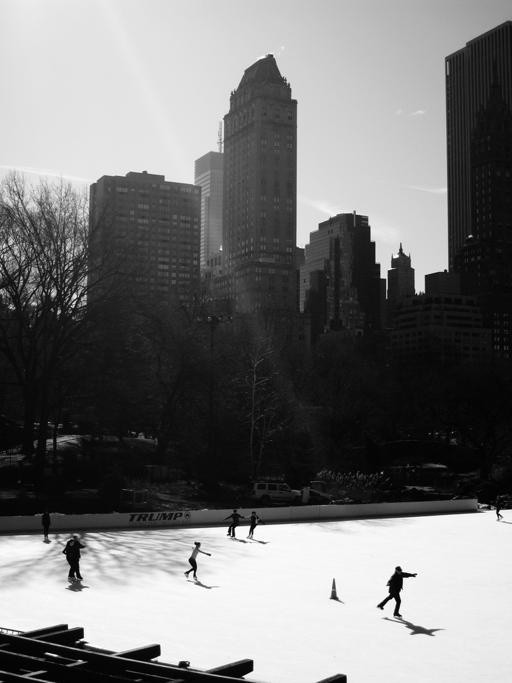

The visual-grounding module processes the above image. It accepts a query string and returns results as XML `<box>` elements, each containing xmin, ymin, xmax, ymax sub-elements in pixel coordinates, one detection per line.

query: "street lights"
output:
<box><xmin>195</xmin><ymin>312</ymin><xmax>232</xmax><ymax>503</ymax></box>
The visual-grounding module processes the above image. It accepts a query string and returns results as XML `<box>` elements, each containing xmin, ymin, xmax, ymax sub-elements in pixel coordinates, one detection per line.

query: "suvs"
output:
<box><xmin>249</xmin><ymin>480</ymin><xmax>302</xmax><ymax>506</ymax></box>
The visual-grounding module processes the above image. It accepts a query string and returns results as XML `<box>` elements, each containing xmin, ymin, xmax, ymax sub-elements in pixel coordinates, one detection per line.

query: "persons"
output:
<box><xmin>62</xmin><ymin>538</ymin><xmax>77</xmax><ymax>582</ymax></box>
<box><xmin>223</xmin><ymin>509</ymin><xmax>244</xmax><ymax>538</ymax></box>
<box><xmin>243</xmin><ymin>510</ymin><xmax>265</xmax><ymax>540</ymax></box>
<box><xmin>41</xmin><ymin>509</ymin><xmax>52</xmax><ymax>542</ymax></box>
<box><xmin>70</xmin><ymin>535</ymin><xmax>87</xmax><ymax>581</ymax></box>
<box><xmin>184</xmin><ymin>540</ymin><xmax>212</xmax><ymax>581</ymax></box>
<box><xmin>494</xmin><ymin>494</ymin><xmax>505</xmax><ymax>521</ymax></box>
<box><xmin>376</xmin><ymin>564</ymin><xmax>418</xmax><ymax>617</ymax></box>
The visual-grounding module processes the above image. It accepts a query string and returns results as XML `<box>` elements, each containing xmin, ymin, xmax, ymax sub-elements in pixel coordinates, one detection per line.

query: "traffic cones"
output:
<box><xmin>330</xmin><ymin>578</ymin><xmax>338</xmax><ymax>599</ymax></box>
